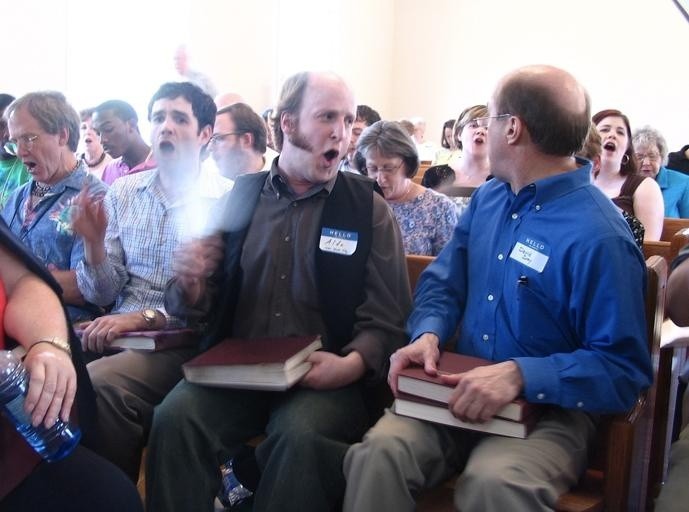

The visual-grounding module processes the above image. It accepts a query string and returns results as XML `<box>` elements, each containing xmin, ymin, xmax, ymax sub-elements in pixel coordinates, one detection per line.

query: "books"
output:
<box><xmin>73</xmin><ymin>326</ymin><xmax>198</xmax><ymax>352</ymax></box>
<box><xmin>392</xmin><ymin>391</ymin><xmax>548</xmax><ymax>441</ymax></box>
<box><xmin>394</xmin><ymin>348</ymin><xmax>547</xmax><ymax>423</ymax></box>
<box><xmin>184</xmin><ymin>333</ymin><xmax>324</xmax><ymax>392</ymax></box>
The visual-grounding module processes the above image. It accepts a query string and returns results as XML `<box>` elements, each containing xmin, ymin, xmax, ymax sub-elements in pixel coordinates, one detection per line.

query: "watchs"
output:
<box><xmin>140</xmin><ymin>309</ymin><xmax>158</xmax><ymax>331</ymax></box>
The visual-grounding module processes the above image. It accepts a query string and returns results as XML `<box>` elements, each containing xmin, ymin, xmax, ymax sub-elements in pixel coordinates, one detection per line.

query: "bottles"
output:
<box><xmin>0</xmin><ymin>349</ymin><xmax>81</xmax><ymax>463</ymax></box>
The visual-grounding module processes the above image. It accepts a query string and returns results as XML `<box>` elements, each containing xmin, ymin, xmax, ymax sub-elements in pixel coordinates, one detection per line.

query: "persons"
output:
<box><xmin>208</xmin><ymin>102</ymin><xmax>279</xmax><ymax>196</ymax></box>
<box><xmin>0</xmin><ymin>215</ymin><xmax>146</xmax><ymax>512</ymax></box>
<box><xmin>72</xmin><ymin>100</ymin><xmax>156</xmax><ymax>189</ymax></box>
<box><xmin>0</xmin><ymin>93</ymin><xmax>34</xmax><ymax>207</ymax></box>
<box><xmin>142</xmin><ymin>67</ymin><xmax>418</xmax><ymax>511</ymax></box>
<box><xmin>337</xmin><ymin>61</ymin><xmax>657</xmax><ymax>512</ymax></box>
<box><xmin>339</xmin><ymin>104</ymin><xmax>494</xmax><ymax>257</ymax></box>
<box><xmin>72</xmin><ymin>80</ymin><xmax>237</xmax><ymax>492</ymax></box>
<box><xmin>587</xmin><ymin>111</ymin><xmax>689</xmax><ymax>243</ymax></box>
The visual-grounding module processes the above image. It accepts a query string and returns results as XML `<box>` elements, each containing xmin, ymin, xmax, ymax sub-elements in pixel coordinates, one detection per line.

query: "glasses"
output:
<box><xmin>361</xmin><ymin>160</ymin><xmax>405</xmax><ymax>176</ymax></box>
<box><xmin>473</xmin><ymin>114</ymin><xmax>511</xmax><ymax>127</ymax></box>
<box><xmin>210</xmin><ymin>133</ymin><xmax>239</xmax><ymax>143</ymax></box>
<box><xmin>635</xmin><ymin>152</ymin><xmax>657</xmax><ymax>161</ymax></box>
<box><xmin>10</xmin><ymin>135</ymin><xmax>38</xmax><ymax>146</ymax></box>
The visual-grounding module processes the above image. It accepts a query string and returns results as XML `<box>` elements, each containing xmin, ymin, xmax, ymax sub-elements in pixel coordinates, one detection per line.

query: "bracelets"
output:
<box><xmin>26</xmin><ymin>335</ymin><xmax>76</xmax><ymax>359</ymax></box>
